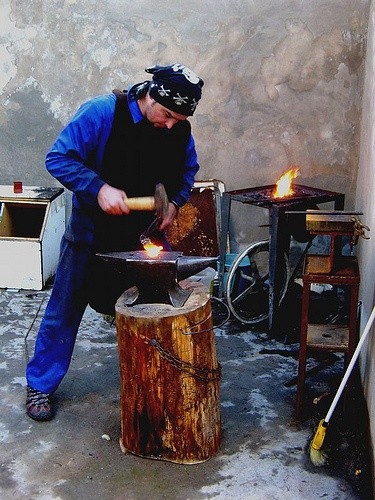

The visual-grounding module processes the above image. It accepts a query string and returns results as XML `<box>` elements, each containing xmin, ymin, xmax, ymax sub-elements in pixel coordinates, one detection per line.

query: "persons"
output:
<box><xmin>25</xmin><ymin>64</ymin><xmax>205</xmax><ymax>420</ymax></box>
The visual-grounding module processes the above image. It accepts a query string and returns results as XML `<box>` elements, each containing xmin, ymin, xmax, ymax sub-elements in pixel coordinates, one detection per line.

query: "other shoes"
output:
<box><xmin>24</xmin><ymin>386</ymin><xmax>55</xmax><ymax>423</ymax></box>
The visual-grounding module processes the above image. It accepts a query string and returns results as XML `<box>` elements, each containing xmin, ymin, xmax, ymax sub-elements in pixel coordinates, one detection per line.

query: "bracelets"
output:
<box><xmin>170</xmin><ymin>201</ymin><xmax>178</xmax><ymax>211</ymax></box>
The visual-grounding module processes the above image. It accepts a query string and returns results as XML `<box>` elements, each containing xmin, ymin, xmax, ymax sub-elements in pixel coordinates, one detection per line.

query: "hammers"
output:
<box><xmin>123</xmin><ymin>182</ymin><xmax>169</xmax><ymax>225</ymax></box>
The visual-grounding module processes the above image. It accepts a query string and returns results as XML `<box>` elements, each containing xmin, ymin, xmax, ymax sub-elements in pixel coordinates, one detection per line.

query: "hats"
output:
<box><xmin>145</xmin><ymin>62</ymin><xmax>204</xmax><ymax>115</ymax></box>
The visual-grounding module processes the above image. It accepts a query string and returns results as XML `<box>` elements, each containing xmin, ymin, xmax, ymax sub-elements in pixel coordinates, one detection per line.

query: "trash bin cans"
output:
<box><xmin>216</xmin><ymin>254</ymin><xmax>253</xmax><ymax>305</ymax></box>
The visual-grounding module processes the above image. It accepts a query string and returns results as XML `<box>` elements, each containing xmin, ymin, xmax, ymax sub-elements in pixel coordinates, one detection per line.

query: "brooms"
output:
<box><xmin>307</xmin><ymin>305</ymin><xmax>375</xmax><ymax>466</ymax></box>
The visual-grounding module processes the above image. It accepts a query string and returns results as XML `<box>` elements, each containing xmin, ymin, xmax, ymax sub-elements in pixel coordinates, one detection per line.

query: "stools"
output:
<box><xmin>115</xmin><ymin>279</ymin><xmax>223</xmax><ymax>465</ymax></box>
<box><xmin>292</xmin><ymin>254</ymin><xmax>362</xmax><ymax>417</ymax></box>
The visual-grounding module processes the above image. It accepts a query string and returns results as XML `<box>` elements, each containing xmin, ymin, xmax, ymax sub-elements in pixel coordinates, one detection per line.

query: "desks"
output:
<box><xmin>0</xmin><ymin>183</ymin><xmax>67</xmax><ymax>291</ymax></box>
